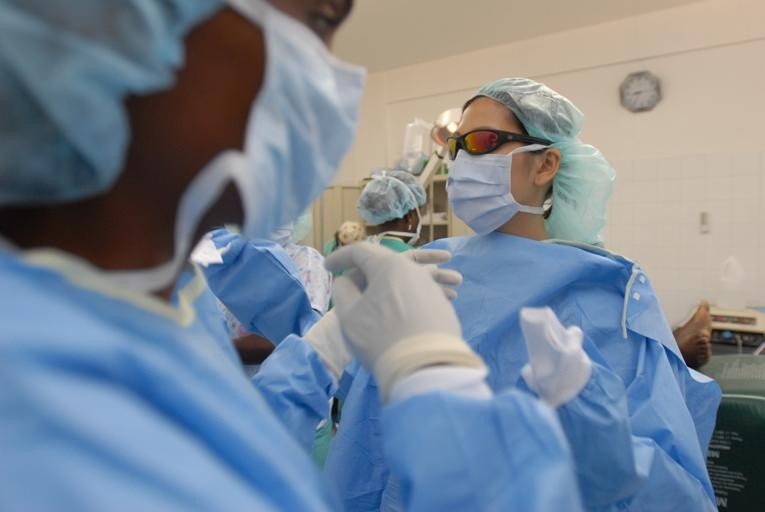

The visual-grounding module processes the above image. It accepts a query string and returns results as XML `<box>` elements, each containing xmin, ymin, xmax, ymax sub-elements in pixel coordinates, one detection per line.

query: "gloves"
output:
<box><xmin>299</xmin><ymin>239</ymin><xmax>490</xmax><ymax>404</ymax></box>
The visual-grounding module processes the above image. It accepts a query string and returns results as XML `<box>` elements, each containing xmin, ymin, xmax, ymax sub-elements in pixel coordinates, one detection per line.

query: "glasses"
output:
<box><xmin>446</xmin><ymin>130</ymin><xmax>556</xmax><ymax>160</ymax></box>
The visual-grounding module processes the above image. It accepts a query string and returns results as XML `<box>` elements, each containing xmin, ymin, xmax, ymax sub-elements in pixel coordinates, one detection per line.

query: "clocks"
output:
<box><xmin>617</xmin><ymin>68</ymin><xmax>663</xmax><ymax>115</ymax></box>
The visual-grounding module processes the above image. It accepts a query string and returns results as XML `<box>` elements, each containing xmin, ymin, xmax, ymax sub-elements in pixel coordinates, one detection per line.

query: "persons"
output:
<box><xmin>670</xmin><ymin>300</ymin><xmax>714</xmax><ymax>369</ymax></box>
<box><xmin>324</xmin><ymin>220</ymin><xmax>365</xmax><ymax>256</ymax></box>
<box><xmin>213</xmin><ymin>213</ymin><xmax>334</xmax><ymax>362</ymax></box>
<box><xmin>328</xmin><ymin>169</ymin><xmax>430</xmax><ymax>419</ymax></box>
<box><xmin>189</xmin><ymin>75</ymin><xmax>727</xmax><ymax>511</ymax></box>
<box><xmin>0</xmin><ymin>0</ymin><xmax>584</xmax><ymax>512</ymax></box>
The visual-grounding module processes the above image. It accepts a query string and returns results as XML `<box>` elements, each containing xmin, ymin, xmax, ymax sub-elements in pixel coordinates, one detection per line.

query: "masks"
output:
<box><xmin>116</xmin><ymin>1</ymin><xmax>366</xmax><ymax>297</ymax></box>
<box><xmin>443</xmin><ymin>142</ymin><xmax>552</xmax><ymax>235</ymax></box>
